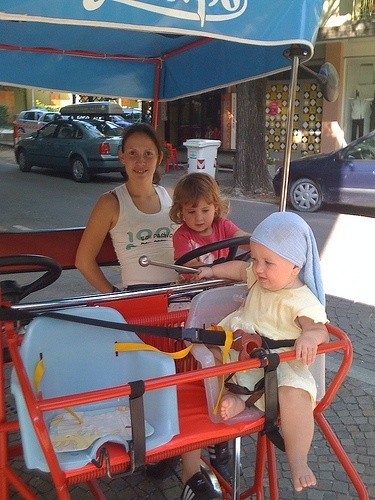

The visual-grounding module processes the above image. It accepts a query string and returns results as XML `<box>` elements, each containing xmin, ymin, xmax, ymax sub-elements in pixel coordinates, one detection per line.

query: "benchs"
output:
<box><xmin>0</xmin><ymin>226</ymin><xmax>375</xmax><ymax>500</ymax></box>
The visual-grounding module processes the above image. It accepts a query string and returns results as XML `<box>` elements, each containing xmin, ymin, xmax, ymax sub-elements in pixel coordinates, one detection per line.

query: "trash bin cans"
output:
<box><xmin>184</xmin><ymin>138</ymin><xmax>222</xmax><ymax>181</ymax></box>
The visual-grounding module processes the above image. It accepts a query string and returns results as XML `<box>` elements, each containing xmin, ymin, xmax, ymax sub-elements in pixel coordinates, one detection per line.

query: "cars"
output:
<box><xmin>14</xmin><ymin>101</ymin><xmax>126</xmax><ymax>184</ymax></box>
<box><xmin>13</xmin><ymin>107</ymin><xmax>152</xmax><ymax>144</ymax></box>
<box><xmin>272</xmin><ymin>129</ymin><xmax>375</xmax><ymax>213</ymax></box>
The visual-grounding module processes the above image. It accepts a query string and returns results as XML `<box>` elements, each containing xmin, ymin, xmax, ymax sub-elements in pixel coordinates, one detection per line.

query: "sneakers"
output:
<box><xmin>181</xmin><ymin>473</ymin><xmax>224</xmax><ymax>500</ymax></box>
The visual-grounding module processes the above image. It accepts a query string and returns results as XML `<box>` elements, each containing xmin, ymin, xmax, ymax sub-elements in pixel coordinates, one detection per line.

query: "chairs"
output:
<box><xmin>61</xmin><ymin>127</ymin><xmax>72</xmax><ymax>136</ymax></box>
<box><xmin>164</xmin><ymin>143</ymin><xmax>178</xmax><ymax>172</ymax></box>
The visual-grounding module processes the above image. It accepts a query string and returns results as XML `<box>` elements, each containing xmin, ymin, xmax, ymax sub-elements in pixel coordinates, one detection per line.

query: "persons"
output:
<box><xmin>74</xmin><ymin>123</ymin><xmax>234</xmax><ymax>500</ymax></box>
<box><xmin>194</xmin><ymin>212</ymin><xmax>329</xmax><ymax>492</ymax></box>
<box><xmin>170</xmin><ymin>171</ymin><xmax>253</xmax><ymax>282</ymax></box>
